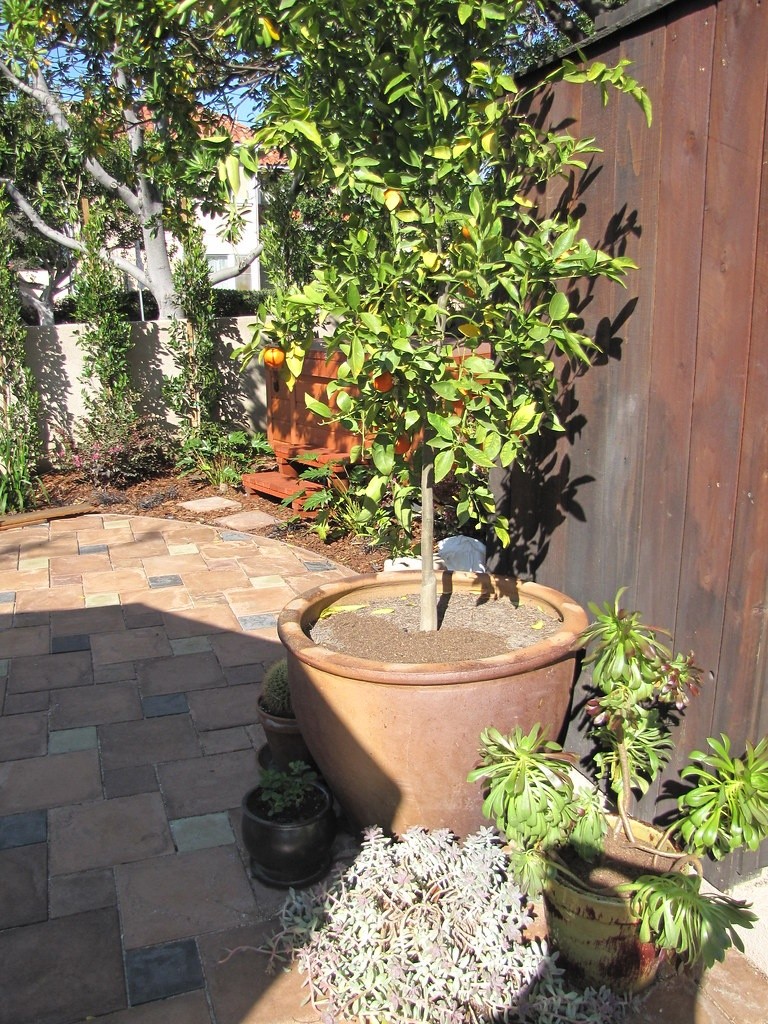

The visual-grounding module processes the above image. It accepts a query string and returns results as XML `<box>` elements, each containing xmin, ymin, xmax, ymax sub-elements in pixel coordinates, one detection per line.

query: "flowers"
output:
<box><xmin>211</xmin><ymin>0</ymin><xmax>654</xmax><ymax>569</ymax></box>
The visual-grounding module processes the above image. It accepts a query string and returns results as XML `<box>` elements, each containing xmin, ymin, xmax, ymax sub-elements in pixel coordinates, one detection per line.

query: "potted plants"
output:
<box><xmin>464</xmin><ymin>585</ymin><xmax>767</xmax><ymax>999</ymax></box>
<box><xmin>240</xmin><ymin>760</ymin><xmax>337</xmax><ymax>888</ymax></box>
<box><xmin>377</xmin><ymin>841</ymin><xmax>551</xmax><ymax>1024</ymax></box>
<box><xmin>256</xmin><ymin>656</ymin><xmax>303</xmax><ymax>760</ymax></box>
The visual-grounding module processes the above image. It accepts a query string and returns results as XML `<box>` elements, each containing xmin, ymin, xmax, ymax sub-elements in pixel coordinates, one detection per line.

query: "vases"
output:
<box><xmin>277</xmin><ymin>568</ymin><xmax>591</xmax><ymax>847</ymax></box>
